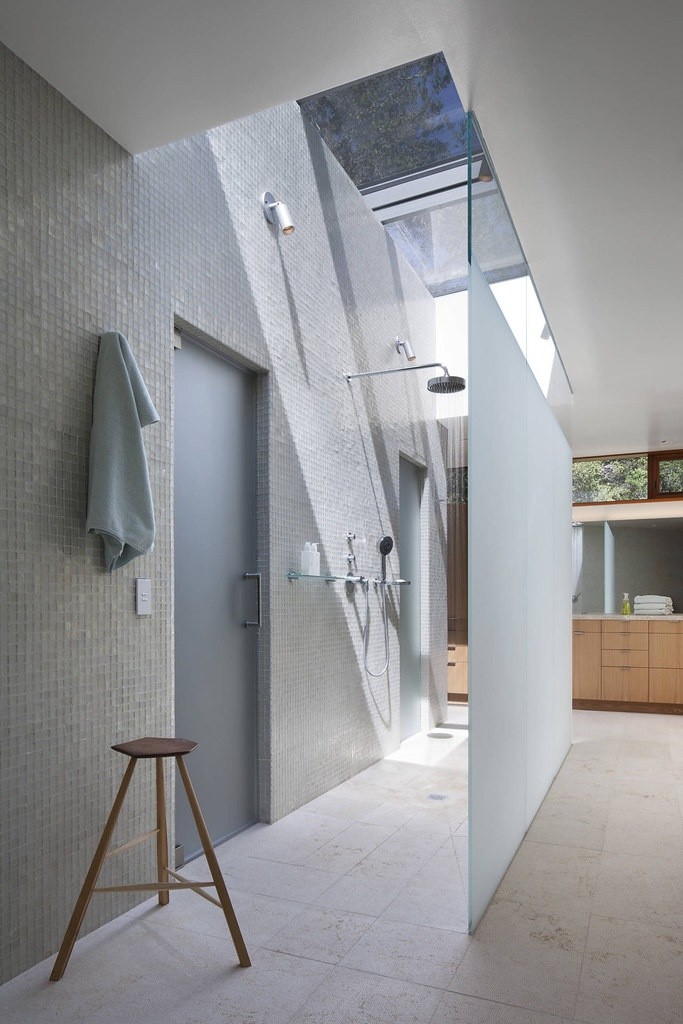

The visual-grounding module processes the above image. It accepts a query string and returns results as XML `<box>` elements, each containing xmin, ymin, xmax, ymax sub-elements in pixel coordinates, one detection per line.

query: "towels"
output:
<box><xmin>85</xmin><ymin>331</ymin><xmax>162</xmax><ymax>574</ymax></box>
<box><xmin>634</xmin><ymin>595</ymin><xmax>675</xmax><ymax>616</ymax></box>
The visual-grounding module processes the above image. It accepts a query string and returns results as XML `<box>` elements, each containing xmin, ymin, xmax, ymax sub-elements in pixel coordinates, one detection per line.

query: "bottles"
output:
<box><xmin>621</xmin><ymin>593</ymin><xmax>631</xmax><ymax>614</ymax></box>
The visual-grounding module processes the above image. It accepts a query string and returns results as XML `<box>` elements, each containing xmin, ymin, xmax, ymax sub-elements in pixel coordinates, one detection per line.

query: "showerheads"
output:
<box><xmin>427</xmin><ymin>373</ymin><xmax>467</xmax><ymax>394</ymax></box>
<box><xmin>376</xmin><ymin>534</ymin><xmax>394</xmax><ymax>581</ymax></box>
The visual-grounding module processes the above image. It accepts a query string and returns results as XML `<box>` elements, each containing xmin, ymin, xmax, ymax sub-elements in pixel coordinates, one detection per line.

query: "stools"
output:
<box><xmin>50</xmin><ymin>737</ymin><xmax>252</xmax><ymax>981</ymax></box>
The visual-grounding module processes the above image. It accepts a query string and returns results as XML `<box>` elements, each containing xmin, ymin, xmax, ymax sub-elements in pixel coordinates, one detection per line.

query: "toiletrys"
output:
<box><xmin>620</xmin><ymin>592</ymin><xmax>631</xmax><ymax>615</ymax></box>
<box><xmin>300</xmin><ymin>541</ymin><xmax>321</xmax><ymax>577</ymax></box>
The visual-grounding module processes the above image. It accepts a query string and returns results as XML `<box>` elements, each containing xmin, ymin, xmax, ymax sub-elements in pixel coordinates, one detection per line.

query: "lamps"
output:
<box><xmin>263</xmin><ymin>191</ymin><xmax>295</xmax><ymax>235</ymax></box>
<box><xmin>396</xmin><ymin>336</ymin><xmax>415</xmax><ymax>361</ymax></box>
<box><xmin>478</xmin><ymin>157</ymin><xmax>493</xmax><ymax>182</ymax></box>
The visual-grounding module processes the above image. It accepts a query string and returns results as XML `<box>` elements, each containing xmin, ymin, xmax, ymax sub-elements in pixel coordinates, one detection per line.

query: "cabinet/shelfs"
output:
<box><xmin>572</xmin><ymin>619</ymin><xmax>601</xmax><ymax>711</ymax></box>
<box><xmin>446</xmin><ymin>619</ymin><xmax>468</xmax><ymax>702</ymax></box>
<box><xmin>601</xmin><ymin>620</ymin><xmax>648</xmax><ymax>713</ymax></box>
<box><xmin>648</xmin><ymin>620</ymin><xmax>683</xmax><ymax>715</ymax></box>
